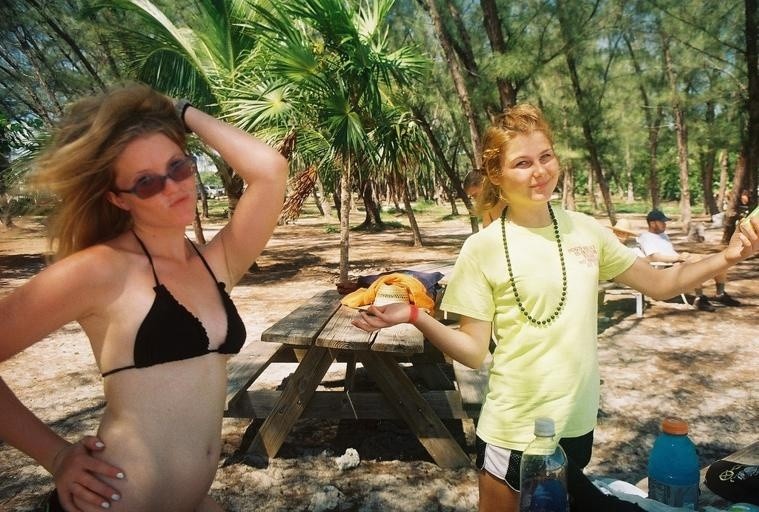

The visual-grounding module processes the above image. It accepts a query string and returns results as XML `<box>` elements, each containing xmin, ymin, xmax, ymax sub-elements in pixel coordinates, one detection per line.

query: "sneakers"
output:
<box><xmin>693</xmin><ymin>295</ymin><xmax>715</xmax><ymax>312</ymax></box>
<box><xmin>714</xmin><ymin>292</ymin><xmax>741</xmax><ymax>307</ymax></box>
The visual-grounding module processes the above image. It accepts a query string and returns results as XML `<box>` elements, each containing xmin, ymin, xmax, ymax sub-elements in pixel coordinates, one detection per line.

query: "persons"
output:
<box><xmin>462</xmin><ymin>168</ymin><xmax>507</xmax><ymax>228</ymax></box>
<box><xmin>349</xmin><ymin>102</ymin><xmax>759</xmax><ymax>510</ymax></box>
<box><xmin>0</xmin><ymin>81</ymin><xmax>291</xmax><ymax>512</ymax></box>
<box><xmin>611</xmin><ymin>219</ymin><xmax>637</xmax><ymax>249</ymax></box>
<box><xmin>728</xmin><ymin>188</ymin><xmax>759</xmax><ymax>243</ymax></box>
<box><xmin>638</xmin><ymin>209</ymin><xmax>741</xmax><ymax>312</ymax></box>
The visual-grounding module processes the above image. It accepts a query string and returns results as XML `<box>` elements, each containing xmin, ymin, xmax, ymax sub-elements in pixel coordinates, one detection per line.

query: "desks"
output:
<box><xmin>242</xmin><ymin>290</ymin><xmax>470</xmax><ymax>469</ymax></box>
<box><xmin>635</xmin><ymin>440</ymin><xmax>759</xmax><ymax>512</ymax></box>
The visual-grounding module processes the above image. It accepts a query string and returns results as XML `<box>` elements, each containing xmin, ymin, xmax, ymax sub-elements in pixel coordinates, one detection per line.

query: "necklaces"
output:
<box><xmin>500</xmin><ymin>201</ymin><xmax>568</xmax><ymax>325</ymax></box>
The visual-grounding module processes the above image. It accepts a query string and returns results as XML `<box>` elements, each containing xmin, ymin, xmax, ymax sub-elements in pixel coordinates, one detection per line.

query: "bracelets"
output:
<box><xmin>175</xmin><ymin>97</ymin><xmax>194</xmax><ymax>135</ymax></box>
<box><xmin>407</xmin><ymin>301</ymin><xmax>422</xmax><ymax>324</ymax></box>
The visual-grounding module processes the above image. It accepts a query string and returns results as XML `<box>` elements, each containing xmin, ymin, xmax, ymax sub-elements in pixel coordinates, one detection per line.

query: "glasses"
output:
<box><xmin>116</xmin><ymin>154</ymin><xmax>198</xmax><ymax>200</ymax></box>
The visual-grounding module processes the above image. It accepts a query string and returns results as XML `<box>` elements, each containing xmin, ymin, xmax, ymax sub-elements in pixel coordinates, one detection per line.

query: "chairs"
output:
<box><xmin>625</xmin><ymin>235</ymin><xmax>700</xmax><ymax>312</ymax></box>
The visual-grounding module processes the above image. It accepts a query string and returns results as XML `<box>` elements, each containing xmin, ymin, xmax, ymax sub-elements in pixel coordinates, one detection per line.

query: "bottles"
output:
<box><xmin>646</xmin><ymin>416</ymin><xmax>702</xmax><ymax>512</ymax></box>
<box><xmin>519</xmin><ymin>417</ymin><xmax>570</xmax><ymax>512</ymax></box>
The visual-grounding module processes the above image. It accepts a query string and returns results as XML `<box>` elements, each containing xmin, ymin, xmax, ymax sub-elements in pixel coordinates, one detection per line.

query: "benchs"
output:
<box><xmin>221</xmin><ymin>338</ymin><xmax>301</xmax><ymax>450</ymax></box>
<box><xmin>421</xmin><ymin>345</ymin><xmax>491</xmax><ymax>440</ymax></box>
<box><xmin>603</xmin><ymin>285</ymin><xmax>645</xmax><ymax>318</ymax></box>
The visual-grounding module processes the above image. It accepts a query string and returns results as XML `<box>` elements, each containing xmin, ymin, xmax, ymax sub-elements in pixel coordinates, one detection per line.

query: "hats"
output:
<box><xmin>351</xmin><ymin>283</ymin><xmax>428</xmax><ymax>311</ymax></box>
<box><xmin>703</xmin><ymin>460</ymin><xmax>759</xmax><ymax>507</ymax></box>
<box><xmin>604</xmin><ymin>219</ymin><xmax>641</xmax><ymax>238</ymax></box>
<box><xmin>646</xmin><ymin>209</ymin><xmax>672</xmax><ymax>222</ymax></box>
